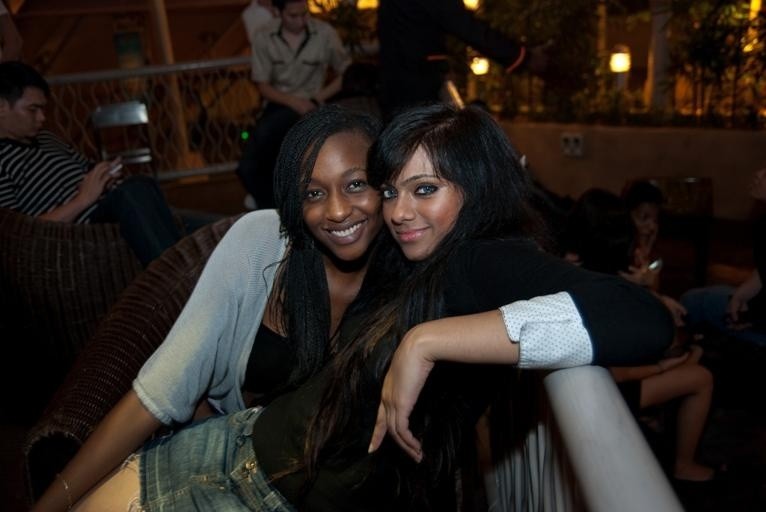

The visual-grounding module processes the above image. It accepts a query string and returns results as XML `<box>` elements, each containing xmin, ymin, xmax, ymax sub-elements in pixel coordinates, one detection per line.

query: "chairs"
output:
<box><xmin>87</xmin><ymin>99</ymin><xmax>160</xmax><ymax>184</ymax></box>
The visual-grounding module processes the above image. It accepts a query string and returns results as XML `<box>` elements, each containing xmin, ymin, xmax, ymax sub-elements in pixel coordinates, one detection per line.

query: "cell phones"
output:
<box><xmin>648</xmin><ymin>259</ymin><xmax>660</xmax><ymax>270</ymax></box>
<box><xmin>109</xmin><ymin>164</ymin><xmax>123</xmax><ymax>175</ymax></box>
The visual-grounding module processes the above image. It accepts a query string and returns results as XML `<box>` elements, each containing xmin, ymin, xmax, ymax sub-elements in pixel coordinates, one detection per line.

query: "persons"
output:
<box><xmin>236</xmin><ymin>1</ymin><xmax>351</xmax><ymax>210</ymax></box>
<box><xmin>30</xmin><ymin>100</ymin><xmax>404</xmax><ymax>510</ymax></box>
<box><xmin>621</xmin><ymin>173</ymin><xmax>753</xmax><ymax>323</ymax></box>
<box><xmin>1</xmin><ymin>59</ymin><xmax>227</xmax><ymax>278</ymax></box>
<box><xmin>374</xmin><ymin>0</ymin><xmax>588</xmax><ymax>125</ymax></box>
<box><xmin>0</xmin><ymin>0</ymin><xmax>22</xmax><ymax>67</ymax></box>
<box><xmin>716</xmin><ymin>158</ymin><xmax>766</xmax><ymax>347</ymax></box>
<box><xmin>63</xmin><ymin>96</ymin><xmax>679</xmax><ymax>509</ymax></box>
<box><xmin>241</xmin><ymin>0</ymin><xmax>286</xmax><ymax>50</ymax></box>
<box><xmin>550</xmin><ymin>188</ymin><xmax>717</xmax><ymax>488</ymax></box>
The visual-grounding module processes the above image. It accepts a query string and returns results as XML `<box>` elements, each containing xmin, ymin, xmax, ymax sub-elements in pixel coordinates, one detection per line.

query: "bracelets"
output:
<box><xmin>55</xmin><ymin>472</ymin><xmax>74</xmax><ymax>510</ymax></box>
